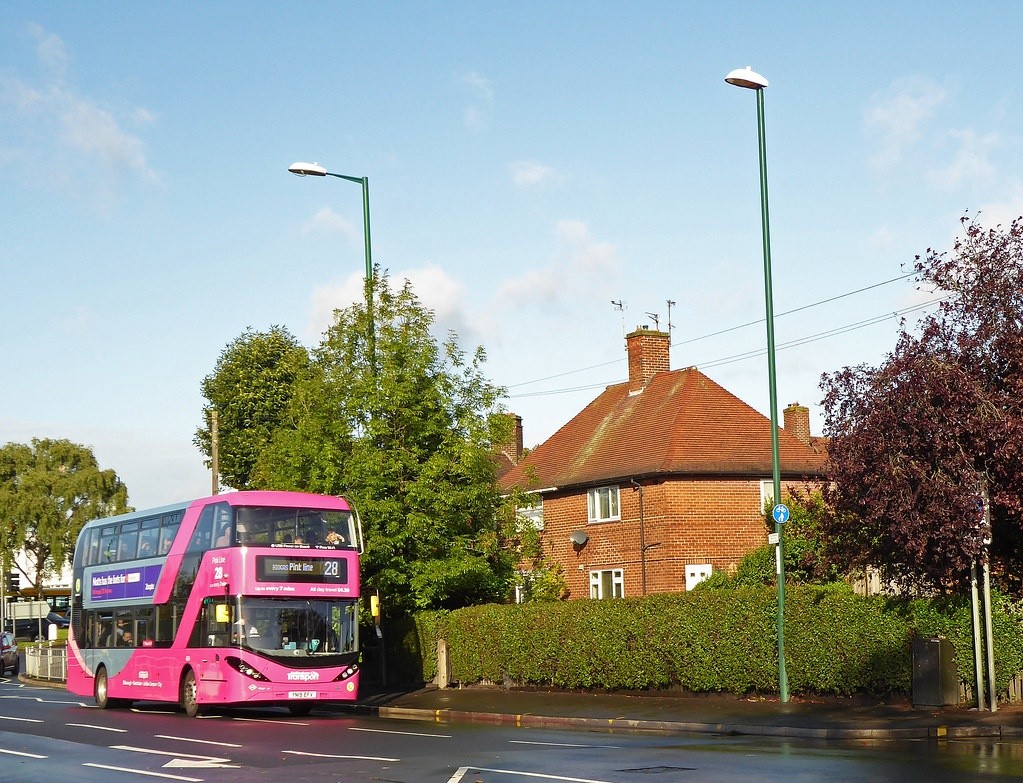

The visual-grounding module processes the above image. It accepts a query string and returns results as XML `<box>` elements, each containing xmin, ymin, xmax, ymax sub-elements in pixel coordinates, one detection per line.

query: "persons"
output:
<box><xmin>117</xmin><ymin>630</ymin><xmax>132</xmax><ymax>648</ymax></box>
<box><xmin>283</xmin><ymin>533</ymin><xmax>303</xmax><ymax>543</ymax></box>
<box><xmin>118</xmin><ymin>523</ymin><xmax>232</xmax><ymax>561</ymax></box>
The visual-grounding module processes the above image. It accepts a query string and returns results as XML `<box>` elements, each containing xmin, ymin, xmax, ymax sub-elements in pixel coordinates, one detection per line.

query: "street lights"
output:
<box><xmin>286</xmin><ymin>161</ymin><xmax>381</xmax><ymax>418</ymax></box>
<box><xmin>724</xmin><ymin>65</ymin><xmax>790</xmax><ymax>703</ymax></box>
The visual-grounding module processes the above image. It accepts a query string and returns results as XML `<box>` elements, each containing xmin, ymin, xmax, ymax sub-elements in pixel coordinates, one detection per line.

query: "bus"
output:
<box><xmin>65</xmin><ymin>489</ymin><xmax>382</xmax><ymax>716</ymax></box>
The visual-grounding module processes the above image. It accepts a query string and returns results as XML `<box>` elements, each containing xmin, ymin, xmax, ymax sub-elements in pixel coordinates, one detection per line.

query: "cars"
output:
<box><xmin>0</xmin><ymin>596</ymin><xmax>77</xmax><ymax>643</ymax></box>
<box><xmin>0</xmin><ymin>631</ymin><xmax>20</xmax><ymax>678</ymax></box>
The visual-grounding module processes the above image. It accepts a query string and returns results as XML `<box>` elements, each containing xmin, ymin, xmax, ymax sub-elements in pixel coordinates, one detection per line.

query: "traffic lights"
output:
<box><xmin>7</xmin><ymin>572</ymin><xmax>20</xmax><ymax>592</ymax></box>
